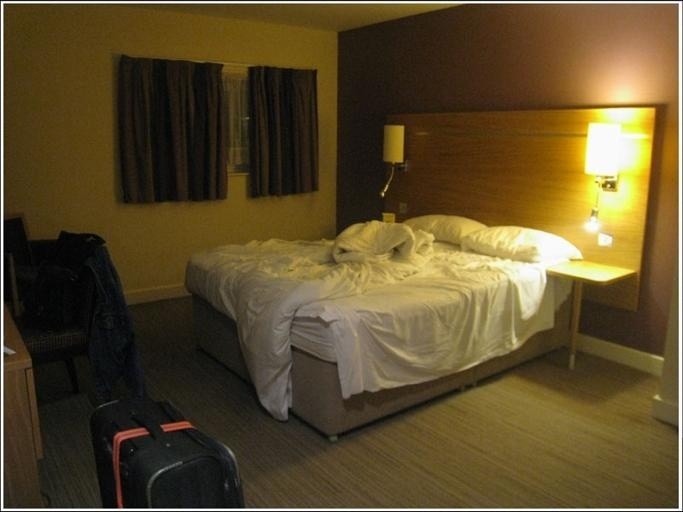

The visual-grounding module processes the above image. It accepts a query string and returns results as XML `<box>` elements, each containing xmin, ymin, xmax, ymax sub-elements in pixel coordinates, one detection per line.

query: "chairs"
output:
<box><xmin>11</xmin><ymin>235</ymin><xmax>106</xmax><ymax>394</ymax></box>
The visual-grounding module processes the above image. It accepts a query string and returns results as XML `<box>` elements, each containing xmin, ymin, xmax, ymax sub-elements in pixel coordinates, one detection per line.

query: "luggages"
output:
<box><xmin>89</xmin><ymin>399</ymin><xmax>244</xmax><ymax>508</ymax></box>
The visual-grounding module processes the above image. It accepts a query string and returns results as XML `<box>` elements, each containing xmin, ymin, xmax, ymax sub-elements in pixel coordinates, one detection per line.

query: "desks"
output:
<box><xmin>546</xmin><ymin>259</ymin><xmax>639</xmax><ymax>369</ymax></box>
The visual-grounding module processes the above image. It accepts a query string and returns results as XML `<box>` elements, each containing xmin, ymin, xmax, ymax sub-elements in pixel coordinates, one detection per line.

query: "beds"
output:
<box><xmin>184</xmin><ymin>226</ymin><xmax>582</xmax><ymax>442</ymax></box>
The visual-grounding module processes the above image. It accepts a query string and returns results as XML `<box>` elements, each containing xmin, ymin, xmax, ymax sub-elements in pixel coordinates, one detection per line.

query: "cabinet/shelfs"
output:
<box><xmin>5</xmin><ymin>307</ymin><xmax>46</xmax><ymax>508</ymax></box>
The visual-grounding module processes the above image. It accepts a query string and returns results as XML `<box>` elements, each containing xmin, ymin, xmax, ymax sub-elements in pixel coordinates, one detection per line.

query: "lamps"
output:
<box><xmin>376</xmin><ymin>124</ymin><xmax>408</xmax><ymax>201</ymax></box>
<box><xmin>583</xmin><ymin>123</ymin><xmax>625</xmax><ymax>233</ymax></box>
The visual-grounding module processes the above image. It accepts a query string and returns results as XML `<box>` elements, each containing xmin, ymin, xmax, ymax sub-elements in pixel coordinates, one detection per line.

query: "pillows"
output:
<box><xmin>460</xmin><ymin>227</ymin><xmax>580</xmax><ymax>268</ymax></box>
<box><xmin>402</xmin><ymin>215</ymin><xmax>489</xmax><ymax>246</ymax></box>
